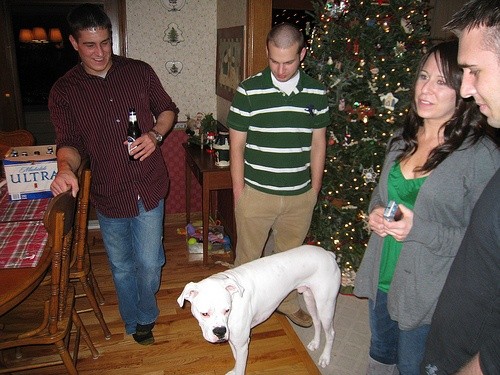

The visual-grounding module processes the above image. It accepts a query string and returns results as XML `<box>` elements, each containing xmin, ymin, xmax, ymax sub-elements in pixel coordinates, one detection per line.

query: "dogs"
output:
<box><xmin>178</xmin><ymin>245</ymin><xmax>341</xmax><ymax>375</ymax></box>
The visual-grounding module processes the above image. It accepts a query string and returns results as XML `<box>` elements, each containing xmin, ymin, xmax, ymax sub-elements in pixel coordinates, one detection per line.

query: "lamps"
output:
<box><xmin>18</xmin><ymin>27</ymin><xmax>63</xmax><ymax>50</ymax></box>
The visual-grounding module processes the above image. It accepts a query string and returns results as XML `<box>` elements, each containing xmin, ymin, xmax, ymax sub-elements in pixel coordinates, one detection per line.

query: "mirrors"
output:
<box><xmin>0</xmin><ymin>0</ymin><xmax>128</xmax><ymax>145</ymax></box>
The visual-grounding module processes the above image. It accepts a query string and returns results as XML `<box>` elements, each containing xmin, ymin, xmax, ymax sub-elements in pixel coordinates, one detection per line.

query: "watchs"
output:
<box><xmin>149</xmin><ymin>128</ymin><xmax>163</xmax><ymax>143</ymax></box>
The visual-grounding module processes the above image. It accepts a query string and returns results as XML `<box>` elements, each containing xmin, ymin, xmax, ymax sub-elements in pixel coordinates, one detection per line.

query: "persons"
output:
<box><xmin>353</xmin><ymin>41</ymin><xmax>500</xmax><ymax>375</ymax></box>
<box><xmin>49</xmin><ymin>6</ymin><xmax>179</xmax><ymax>346</ymax></box>
<box><xmin>226</xmin><ymin>23</ymin><xmax>331</xmax><ymax>267</ymax></box>
<box><xmin>419</xmin><ymin>0</ymin><xmax>500</xmax><ymax>375</ymax></box>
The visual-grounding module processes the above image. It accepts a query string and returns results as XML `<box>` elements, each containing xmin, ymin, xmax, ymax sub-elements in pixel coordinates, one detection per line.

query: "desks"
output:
<box><xmin>182</xmin><ymin>142</ymin><xmax>237</xmax><ymax>264</ymax></box>
<box><xmin>0</xmin><ymin>167</ymin><xmax>52</xmax><ymax>317</ymax></box>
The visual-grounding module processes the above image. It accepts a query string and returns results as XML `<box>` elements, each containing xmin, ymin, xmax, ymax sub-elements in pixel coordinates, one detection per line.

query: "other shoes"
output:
<box><xmin>132</xmin><ymin>322</ymin><xmax>155</xmax><ymax>345</ymax></box>
<box><xmin>275</xmin><ymin>309</ymin><xmax>313</xmax><ymax>328</ymax></box>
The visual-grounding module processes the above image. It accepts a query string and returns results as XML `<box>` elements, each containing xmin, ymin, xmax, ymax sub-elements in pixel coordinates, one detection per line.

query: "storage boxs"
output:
<box><xmin>3</xmin><ymin>144</ymin><xmax>58</xmax><ymax>200</ymax></box>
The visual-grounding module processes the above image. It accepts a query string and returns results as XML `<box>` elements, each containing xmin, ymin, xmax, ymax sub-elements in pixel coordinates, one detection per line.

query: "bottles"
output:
<box><xmin>126</xmin><ymin>108</ymin><xmax>142</xmax><ymax>160</ymax></box>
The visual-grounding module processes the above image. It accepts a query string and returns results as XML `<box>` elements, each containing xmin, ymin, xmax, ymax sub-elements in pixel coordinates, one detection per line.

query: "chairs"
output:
<box><xmin>0</xmin><ymin>153</ymin><xmax>111</xmax><ymax>375</ymax></box>
<box><xmin>0</xmin><ymin>128</ymin><xmax>35</xmax><ymax>157</ymax></box>
